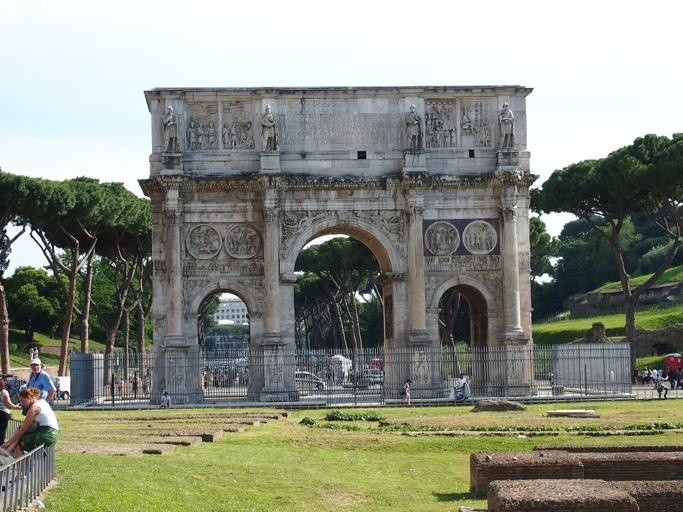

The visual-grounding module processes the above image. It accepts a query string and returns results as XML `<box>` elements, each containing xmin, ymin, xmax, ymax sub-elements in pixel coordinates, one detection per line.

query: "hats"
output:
<box><xmin>31</xmin><ymin>358</ymin><xmax>41</xmax><ymax>365</ymax></box>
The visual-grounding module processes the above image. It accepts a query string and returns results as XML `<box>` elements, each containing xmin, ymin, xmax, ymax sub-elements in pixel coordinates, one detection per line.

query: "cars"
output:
<box><xmin>294</xmin><ymin>370</ymin><xmax>325</xmax><ymax>392</ymax></box>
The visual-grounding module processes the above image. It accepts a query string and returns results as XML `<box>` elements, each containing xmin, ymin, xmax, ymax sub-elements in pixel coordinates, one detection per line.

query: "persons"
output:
<box><xmin>260</xmin><ymin>103</ymin><xmax>280</xmax><ymax>152</ymax></box>
<box><xmin>427</xmin><ymin>112</ymin><xmax>491</xmax><ymax>147</ymax></box>
<box><xmin>0</xmin><ymin>388</ymin><xmax>58</xmax><ymax>484</ymax></box>
<box><xmin>54</xmin><ymin>377</ymin><xmax>60</xmax><ymax>399</ymax></box>
<box><xmin>26</xmin><ymin>358</ymin><xmax>56</xmax><ymax>402</ymax></box>
<box><xmin>633</xmin><ymin>364</ymin><xmax>682</xmax><ymax>400</ymax></box>
<box><xmin>0</xmin><ymin>373</ymin><xmax>20</xmax><ymax>445</ymax></box>
<box><xmin>497</xmin><ymin>102</ymin><xmax>515</xmax><ymax>149</ymax></box>
<box><xmin>28</xmin><ymin>345</ymin><xmax>33</xmax><ymax>360</ymax></box>
<box><xmin>161</xmin><ymin>104</ymin><xmax>177</xmax><ymax>152</ymax></box>
<box><xmin>185</xmin><ymin>120</ymin><xmax>252</xmax><ymax>149</ymax></box>
<box><xmin>453</xmin><ymin>374</ymin><xmax>471</xmax><ymax>401</ymax></box>
<box><xmin>33</xmin><ymin>346</ymin><xmax>38</xmax><ymax>358</ymax></box>
<box><xmin>159</xmin><ymin>391</ymin><xmax>170</xmax><ymax>408</ymax></box>
<box><xmin>19</xmin><ymin>380</ymin><xmax>26</xmax><ymax>392</ymax></box>
<box><xmin>402</xmin><ymin>102</ymin><xmax>422</xmax><ymax>149</ymax></box>
<box><xmin>400</xmin><ymin>378</ymin><xmax>411</xmax><ymax>405</ymax></box>
<box><xmin>201</xmin><ymin>363</ymin><xmax>228</xmax><ymax>389</ymax></box>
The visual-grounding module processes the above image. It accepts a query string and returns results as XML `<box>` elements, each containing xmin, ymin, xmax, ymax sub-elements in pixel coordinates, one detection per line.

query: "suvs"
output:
<box><xmin>363</xmin><ymin>368</ymin><xmax>384</xmax><ymax>386</ymax></box>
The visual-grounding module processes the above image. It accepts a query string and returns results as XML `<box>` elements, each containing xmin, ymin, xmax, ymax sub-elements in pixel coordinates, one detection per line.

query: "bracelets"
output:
<box><xmin>44</xmin><ymin>399</ymin><xmax>49</xmax><ymax>403</ymax></box>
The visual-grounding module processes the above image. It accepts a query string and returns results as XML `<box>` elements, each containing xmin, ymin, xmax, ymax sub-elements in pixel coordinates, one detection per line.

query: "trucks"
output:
<box><xmin>19</xmin><ymin>375</ymin><xmax>72</xmax><ymax>400</ymax></box>
